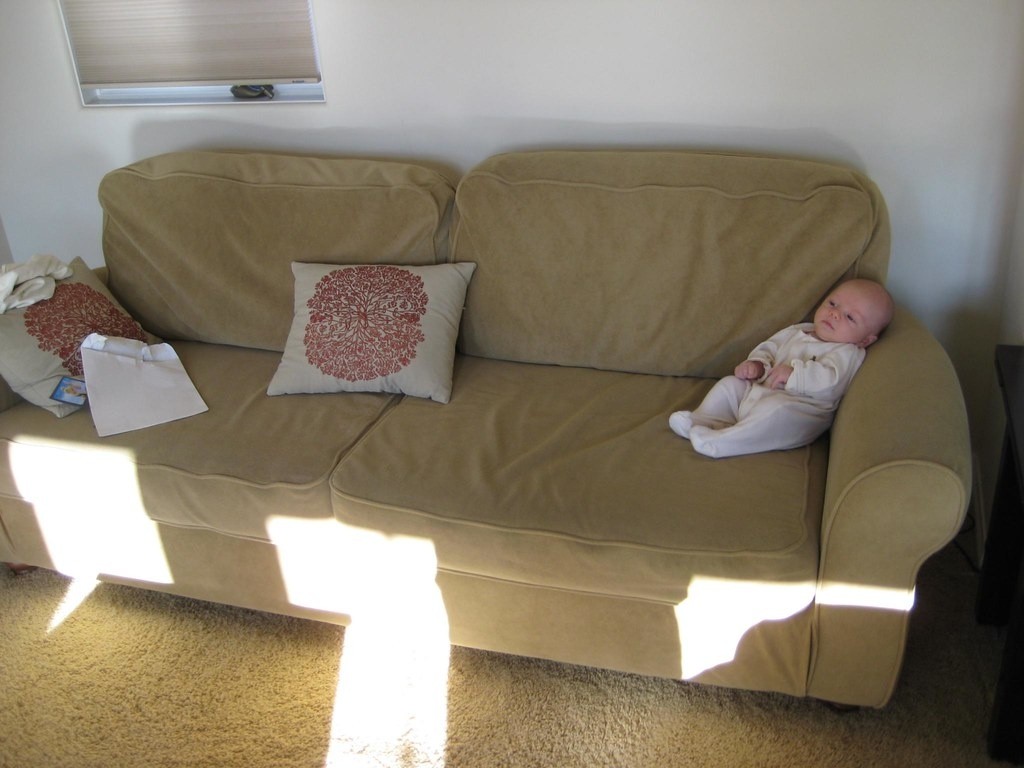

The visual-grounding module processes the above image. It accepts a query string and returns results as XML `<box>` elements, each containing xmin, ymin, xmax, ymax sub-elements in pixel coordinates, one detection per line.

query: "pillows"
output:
<box><xmin>266</xmin><ymin>259</ymin><xmax>478</xmax><ymax>405</ymax></box>
<box><xmin>0</xmin><ymin>254</ymin><xmax>165</xmax><ymax>420</ymax></box>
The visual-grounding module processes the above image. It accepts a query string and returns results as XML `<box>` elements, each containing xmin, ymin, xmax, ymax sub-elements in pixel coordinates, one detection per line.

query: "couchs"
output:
<box><xmin>0</xmin><ymin>148</ymin><xmax>974</xmax><ymax>717</ymax></box>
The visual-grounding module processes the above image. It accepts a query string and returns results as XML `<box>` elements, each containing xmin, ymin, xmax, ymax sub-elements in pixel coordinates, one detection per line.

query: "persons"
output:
<box><xmin>667</xmin><ymin>277</ymin><xmax>895</xmax><ymax>460</ymax></box>
